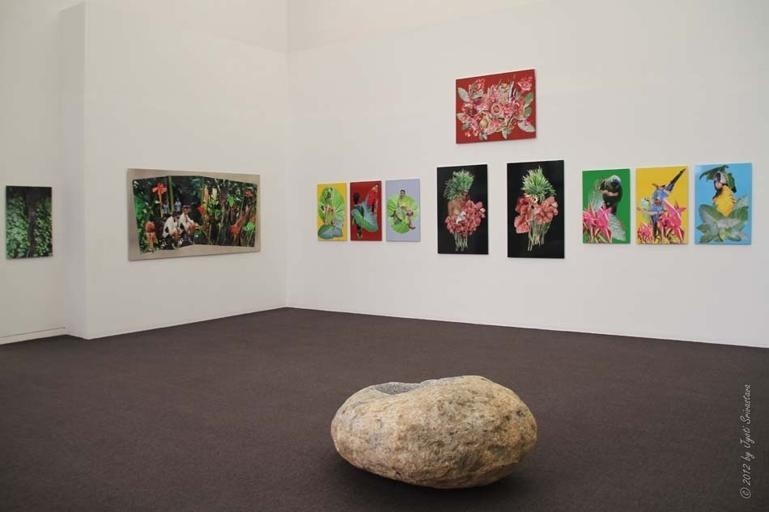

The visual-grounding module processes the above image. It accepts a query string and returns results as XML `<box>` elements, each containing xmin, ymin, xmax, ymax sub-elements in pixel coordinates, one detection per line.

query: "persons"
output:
<box><xmin>397</xmin><ymin>190</ymin><xmax>416</xmax><ymax>229</ymax></box>
<box><xmin>366</xmin><ymin>183</ymin><xmax>379</xmax><ymax>215</ymax></box>
<box><xmin>145</xmin><ymin>198</ymin><xmax>199</xmax><ymax>250</ymax></box>
<box><xmin>351</xmin><ymin>192</ymin><xmax>364</xmax><ymax>240</ymax></box>
